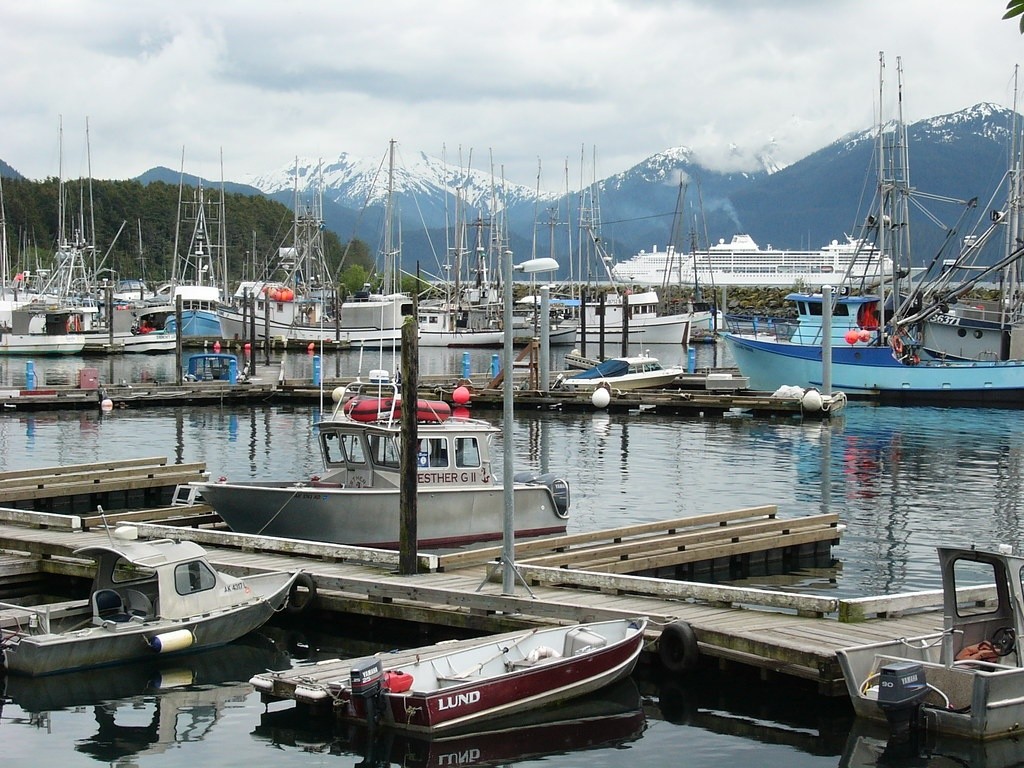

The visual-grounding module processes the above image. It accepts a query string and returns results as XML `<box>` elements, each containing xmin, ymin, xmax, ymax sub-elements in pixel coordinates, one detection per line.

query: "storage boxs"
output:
<box><xmin>562</xmin><ymin>625</ymin><xmax>607</xmax><ymax>656</ymax></box>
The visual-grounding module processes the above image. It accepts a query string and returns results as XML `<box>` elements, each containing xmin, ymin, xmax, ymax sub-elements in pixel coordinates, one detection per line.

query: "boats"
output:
<box><xmin>332</xmin><ymin>616</ymin><xmax>652</xmax><ymax>741</ymax></box>
<box><xmin>186</xmin><ymin>263</ymin><xmax>569</xmax><ymax>550</ymax></box>
<box><xmin>0</xmin><ymin>506</ymin><xmax>308</xmax><ymax>675</ymax></box>
<box><xmin>834</xmin><ymin>543</ymin><xmax>1024</xmax><ymax>742</ymax></box>
<box><xmin>560</xmin><ymin>355</ymin><xmax>682</xmax><ymax>394</ymax></box>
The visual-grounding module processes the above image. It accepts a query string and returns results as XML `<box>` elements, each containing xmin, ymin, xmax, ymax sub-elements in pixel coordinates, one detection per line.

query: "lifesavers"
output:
<box><xmin>658</xmin><ymin>676</ymin><xmax>697</xmax><ymax>727</ymax></box>
<box><xmin>657</xmin><ymin>622</ymin><xmax>698</xmax><ymax>674</ymax></box>
<box><xmin>65</xmin><ymin>315</ymin><xmax>81</xmax><ymax>333</ymax></box>
<box><xmin>893</xmin><ymin>336</ymin><xmax>903</xmax><ymax>353</ymax></box>
<box><xmin>344</xmin><ymin>393</ymin><xmax>449</xmax><ymax>426</ymax></box>
<box><xmin>286</xmin><ymin>574</ymin><xmax>318</xmax><ymax>613</ymax></box>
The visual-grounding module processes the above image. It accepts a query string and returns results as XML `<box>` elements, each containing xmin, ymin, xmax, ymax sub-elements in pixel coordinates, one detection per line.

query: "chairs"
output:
<box><xmin>126</xmin><ymin>590</ymin><xmax>153</xmax><ymax>621</ymax></box>
<box><xmin>91</xmin><ymin>589</ymin><xmax>132</xmax><ymax>627</ymax></box>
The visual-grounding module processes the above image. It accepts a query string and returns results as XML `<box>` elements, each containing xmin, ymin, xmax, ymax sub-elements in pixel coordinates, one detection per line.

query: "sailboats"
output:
<box><xmin>723</xmin><ymin>52</ymin><xmax>1024</xmax><ymax>403</ymax></box>
<box><xmin>0</xmin><ymin>112</ymin><xmax>722</xmax><ymax>352</ymax></box>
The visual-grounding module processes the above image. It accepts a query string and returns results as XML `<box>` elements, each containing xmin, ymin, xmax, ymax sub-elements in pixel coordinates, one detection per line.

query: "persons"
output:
<box><xmin>139</xmin><ymin>318</ymin><xmax>155</xmax><ymax>335</ymax></box>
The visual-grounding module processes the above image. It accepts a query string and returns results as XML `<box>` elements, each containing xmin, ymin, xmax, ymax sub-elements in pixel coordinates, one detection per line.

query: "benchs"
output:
<box><xmin>438</xmin><ymin>675</ymin><xmax>479</xmax><ymax>688</ymax></box>
<box><xmin>506</xmin><ymin>659</ymin><xmax>533</xmax><ymax>671</ymax></box>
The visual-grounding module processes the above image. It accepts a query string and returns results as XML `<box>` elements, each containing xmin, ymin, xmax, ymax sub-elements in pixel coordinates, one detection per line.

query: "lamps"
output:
<box><xmin>114</xmin><ymin>526</ymin><xmax>139</xmax><ymax>546</ymax></box>
